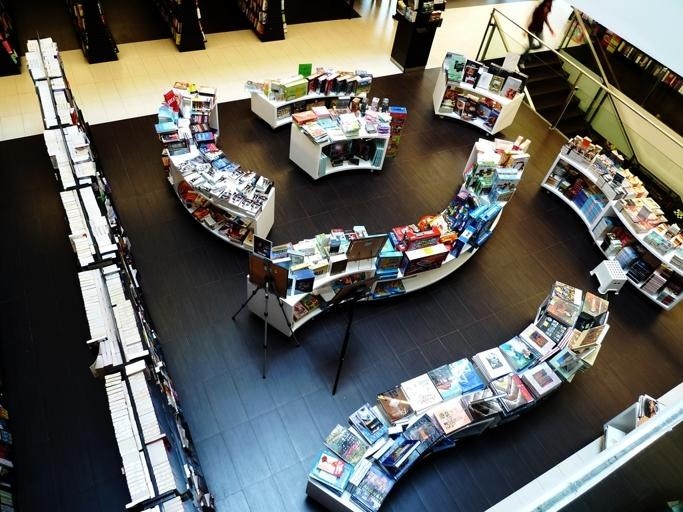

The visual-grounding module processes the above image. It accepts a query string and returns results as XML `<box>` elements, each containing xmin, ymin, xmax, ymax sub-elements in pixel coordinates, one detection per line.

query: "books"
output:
<box><xmin>540</xmin><ymin>132</ymin><xmax>682</xmax><ymax>308</ymax></box>
<box><xmin>79</xmin><ymin>264</ymin><xmax>216</xmax><ymax>512</ymax></box>
<box><xmin>243</xmin><ymin>60</ymin><xmax>392</xmax><ymax>177</ymax></box>
<box><xmin>562</xmin><ymin>10</ymin><xmax>682</xmax><ymax>97</ymax></box>
<box><xmin>236</xmin><ymin>0</ymin><xmax>289</xmax><ymax>35</ymax></box>
<box><xmin>600</xmin><ymin>394</ymin><xmax>665</xmax><ymax>452</ymax></box>
<box><xmin>69</xmin><ymin>0</ymin><xmax>119</xmax><ymax>56</ymax></box>
<box><xmin>153</xmin><ymin>77</ymin><xmax>274</xmax><ymax>250</ymax></box>
<box><xmin>269</xmin><ymin>225</ymin><xmax>406</xmax><ymax>326</ymax></box>
<box><xmin>157</xmin><ymin>0</ymin><xmax>206</xmax><ymax>45</ymax></box>
<box><xmin>396</xmin><ymin>0</ymin><xmax>446</xmax><ymax>23</ymax></box>
<box><xmin>390</xmin><ymin>134</ymin><xmax>532</xmax><ymax>277</ymax></box>
<box><xmin>0</xmin><ymin>396</ymin><xmax>18</xmax><ymax>512</ymax></box>
<box><xmin>437</xmin><ymin>50</ymin><xmax>528</xmax><ymax>132</ymax></box>
<box><xmin>0</xmin><ymin>8</ymin><xmax>18</xmax><ymax>67</ymax></box>
<box><xmin>305</xmin><ymin>355</ymin><xmax>509</xmax><ymax>511</ymax></box>
<box><xmin>42</xmin><ymin>127</ymin><xmax>132</xmax><ymax>267</ymax></box>
<box><xmin>472</xmin><ymin>279</ymin><xmax>612</xmax><ymax>415</ymax></box>
<box><xmin>26</xmin><ymin>37</ymin><xmax>88</xmax><ymax>130</ymax></box>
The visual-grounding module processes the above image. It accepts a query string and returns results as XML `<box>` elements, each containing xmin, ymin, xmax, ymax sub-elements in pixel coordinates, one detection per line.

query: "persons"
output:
<box><xmin>517</xmin><ymin>0</ymin><xmax>555</xmax><ymax>69</ymax></box>
